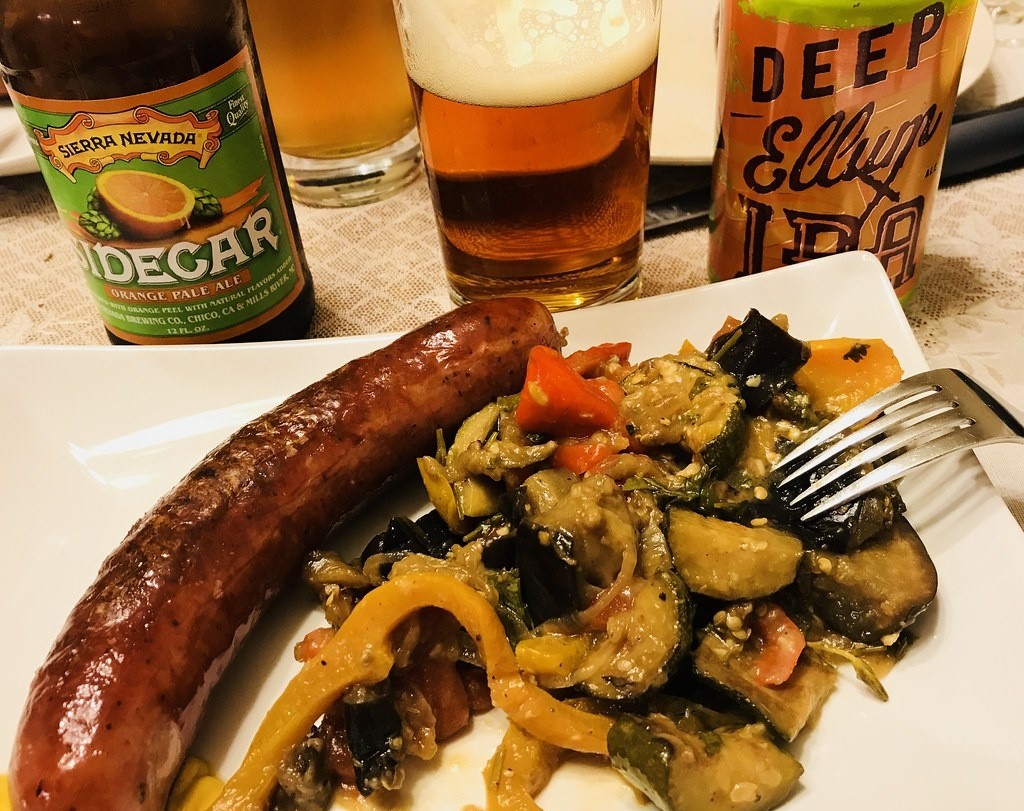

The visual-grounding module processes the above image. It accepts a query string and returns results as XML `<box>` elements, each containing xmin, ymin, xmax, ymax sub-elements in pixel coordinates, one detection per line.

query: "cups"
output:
<box><xmin>239</xmin><ymin>2</ymin><xmax>418</xmax><ymax>216</ymax></box>
<box><xmin>391</xmin><ymin>0</ymin><xmax>661</xmax><ymax>340</ymax></box>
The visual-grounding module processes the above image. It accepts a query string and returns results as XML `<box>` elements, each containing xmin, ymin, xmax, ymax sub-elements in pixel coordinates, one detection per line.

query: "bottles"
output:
<box><xmin>706</xmin><ymin>1</ymin><xmax>980</xmax><ymax>318</ymax></box>
<box><xmin>2</xmin><ymin>2</ymin><xmax>317</xmax><ymax>370</ymax></box>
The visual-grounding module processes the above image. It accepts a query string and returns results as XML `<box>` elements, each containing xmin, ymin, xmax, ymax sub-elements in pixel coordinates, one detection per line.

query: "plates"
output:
<box><xmin>0</xmin><ymin>245</ymin><xmax>1024</xmax><ymax>810</ymax></box>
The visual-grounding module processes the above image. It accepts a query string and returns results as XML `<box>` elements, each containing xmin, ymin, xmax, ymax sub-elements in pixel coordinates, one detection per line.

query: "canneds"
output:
<box><xmin>0</xmin><ymin>1</ymin><xmax>316</xmax><ymax>345</ymax></box>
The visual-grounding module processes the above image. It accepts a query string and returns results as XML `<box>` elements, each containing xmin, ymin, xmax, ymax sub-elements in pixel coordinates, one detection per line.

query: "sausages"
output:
<box><xmin>8</xmin><ymin>299</ymin><xmax>567</xmax><ymax>811</ymax></box>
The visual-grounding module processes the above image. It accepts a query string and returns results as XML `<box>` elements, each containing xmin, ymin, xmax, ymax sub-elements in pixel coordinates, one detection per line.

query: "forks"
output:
<box><xmin>768</xmin><ymin>368</ymin><xmax>1024</xmax><ymax>526</ymax></box>
